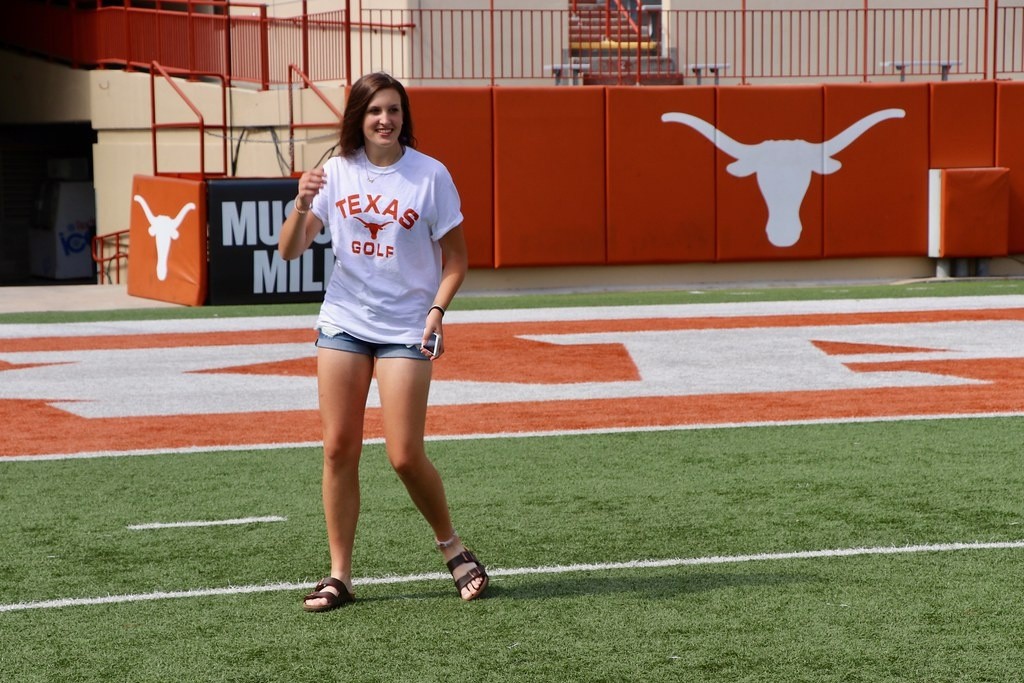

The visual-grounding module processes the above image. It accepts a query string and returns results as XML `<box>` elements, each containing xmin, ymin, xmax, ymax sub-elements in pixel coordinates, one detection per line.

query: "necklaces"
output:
<box><xmin>365</xmin><ymin>150</ymin><xmax>402</xmax><ymax>183</ymax></box>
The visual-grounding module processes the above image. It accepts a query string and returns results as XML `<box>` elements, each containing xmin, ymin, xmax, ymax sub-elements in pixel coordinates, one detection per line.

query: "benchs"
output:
<box><xmin>544</xmin><ymin>64</ymin><xmax>590</xmax><ymax>86</ymax></box>
<box><xmin>685</xmin><ymin>63</ymin><xmax>730</xmax><ymax>84</ymax></box>
<box><xmin>880</xmin><ymin>59</ymin><xmax>962</xmax><ymax>82</ymax></box>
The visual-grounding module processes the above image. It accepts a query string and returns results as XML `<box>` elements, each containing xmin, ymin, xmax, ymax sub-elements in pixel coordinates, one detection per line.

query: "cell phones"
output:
<box><xmin>421</xmin><ymin>328</ymin><xmax>441</xmax><ymax>357</ymax></box>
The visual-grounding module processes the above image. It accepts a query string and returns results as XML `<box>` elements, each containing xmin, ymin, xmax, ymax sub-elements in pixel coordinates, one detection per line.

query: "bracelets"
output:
<box><xmin>428</xmin><ymin>305</ymin><xmax>444</xmax><ymax>316</ymax></box>
<box><xmin>294</xmin><ymin>194</ymin><xmax>313</xmax><ymax>214</ymax></box>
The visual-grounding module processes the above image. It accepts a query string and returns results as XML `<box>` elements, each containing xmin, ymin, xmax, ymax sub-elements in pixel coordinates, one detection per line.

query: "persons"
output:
<box><xmin>278</xmin><ymin>71</ymin><xmax>490</xmax><ymax>611</ymax></box>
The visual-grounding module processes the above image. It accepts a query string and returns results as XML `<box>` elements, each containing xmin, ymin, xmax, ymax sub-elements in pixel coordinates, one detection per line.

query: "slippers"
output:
<box><xmin>302</xmin><ymin>577</ymin><xmax>356</xmax><ymax>612</ymax></box>
<box><xmin>447</xmin><ymin>545</ymin><xmax>489</xmax><ymax>599</ymax></box>
